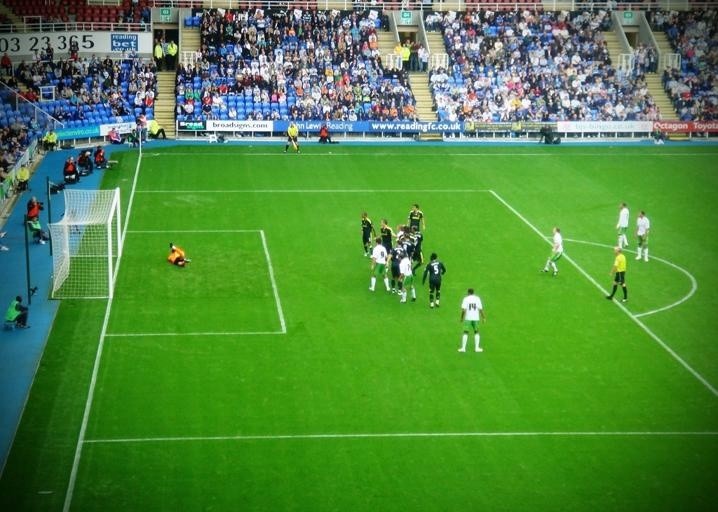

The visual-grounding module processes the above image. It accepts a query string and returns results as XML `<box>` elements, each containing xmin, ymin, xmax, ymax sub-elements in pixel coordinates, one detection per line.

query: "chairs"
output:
<box><xmin>179</xmin><ymin>0</ymin><xmax>716</xmax><ymax>138</ymax></box>
<box><xmin>1</xmin><ymin>3</ymin><xmax>179</xmax><ymax>193</ymax></box>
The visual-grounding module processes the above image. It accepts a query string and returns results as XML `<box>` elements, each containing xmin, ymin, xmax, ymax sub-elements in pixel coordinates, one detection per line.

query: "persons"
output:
<box><xmin>175</xmin><ymin>1</ymin><xmax>429</xmax><ymax>153</ymax></box>
<box><xmin>540</xmin><ymin>227</ymin><xmax>563</xmax><ymax>276</ymax></box>
<box><xmin>423</xmin><ymin>1</ymin><xmax>670</xmax><ymax>144</ymax></box>
<box><xmin>1</xmin><ymin>1</ymin><xmax>191</xmax><ymax>329</ymax></box>
<box><xmin>457</xmin><ymin>288</ymin><xmax>486</xmax><ymax>352</ymax></box>
<box><xmin>647</xmin><ymin>1</ymin><xmax>718</xmax><ymax>138</ymax></box>
<box><xmin>604</xmin><ymin>202</ymin><xmax>650</xmax><ymax>302</ymax></box>
<box><xmin>362</xmin><ymin>204</ymin><xmax>446</xmax><ymax>309</ymax></box>
